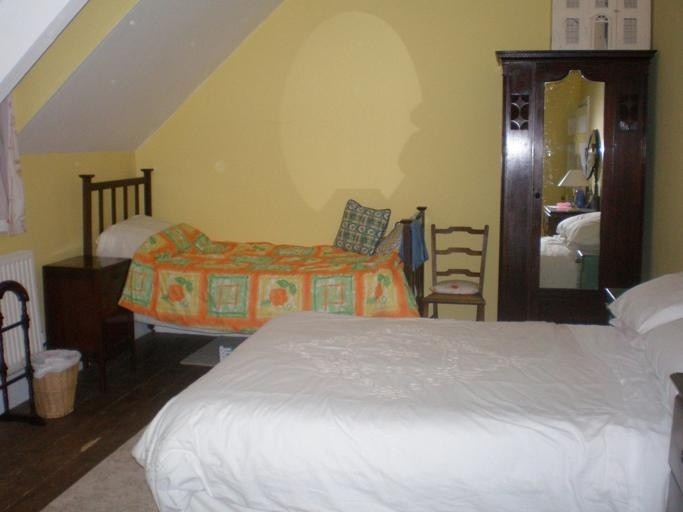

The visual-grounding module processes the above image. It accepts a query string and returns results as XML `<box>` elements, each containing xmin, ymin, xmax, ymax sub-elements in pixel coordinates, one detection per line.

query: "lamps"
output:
<box><xmin>557</xmin><ymin>169</ymin><xmax>592</xmax><ymax>205</ymax></box>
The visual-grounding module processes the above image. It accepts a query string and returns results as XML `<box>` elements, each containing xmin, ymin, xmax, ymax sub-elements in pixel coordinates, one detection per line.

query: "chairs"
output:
<box><xmin>419</xmin><ymin>223</ymin><xmax>489</xmax><ymax>321</ymax></box>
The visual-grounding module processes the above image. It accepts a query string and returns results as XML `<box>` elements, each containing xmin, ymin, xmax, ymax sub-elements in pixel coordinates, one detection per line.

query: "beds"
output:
<box><xmin>80</xmin><ymin>166</ymin><xmax>427</xmax><ymax>341</ymax></box>
<box><xmin>131</xmin><ymin>310</ymin><xmax>675</xmax><ymax>511</ymax></box>
<box><xmin>539</xmin><ymin>235</ymin><xmax>580</xmax><ymax>290</ymax></box>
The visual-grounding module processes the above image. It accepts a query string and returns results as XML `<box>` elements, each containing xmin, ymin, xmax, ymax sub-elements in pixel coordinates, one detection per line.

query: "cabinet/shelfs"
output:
<box><xmin>496</xmin><ymin>44</ymin><xmax>660</xmax><ymax>325</ymax></box>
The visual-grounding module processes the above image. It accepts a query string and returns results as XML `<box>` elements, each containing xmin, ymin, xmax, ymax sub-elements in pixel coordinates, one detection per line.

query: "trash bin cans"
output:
<box><xmin>22</xmin><ymin>349</ymin><xmax>82</xmax><ymax>419</ymax></box>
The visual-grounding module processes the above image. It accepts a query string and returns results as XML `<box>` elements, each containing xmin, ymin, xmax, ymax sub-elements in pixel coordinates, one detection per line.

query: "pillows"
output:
<box><xmin>332</xmin><ymin>199</ymin><xmax>390</xmax><ymax>256</ymax></box>
<box><xmin>555</xmin><ymin>210</ymin><xmax>600</xmax><ymax>250</ymax></box>
<box><xmin>428</xmin><ymin>278</ymin><xmax>480</xmax><ymax>295</ymax></box>
<box><xmin>96</xmin><ymin>215</ymin><xmax>174</xmax><ymax>261</ymax></box>
<box><xmin>374</xmin><ymin>221</ymin><xmax>404</xmax><ymax>256</ymax></box>
<box><xmin>605</xmin><ymin>271</ymin><xmax>683</xmax><ymax>400</ymax></box>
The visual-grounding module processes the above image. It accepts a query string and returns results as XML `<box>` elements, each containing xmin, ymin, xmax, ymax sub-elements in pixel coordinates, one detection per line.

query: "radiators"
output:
<box><xmin>0</xmin><ymin>250</ymin><xmax>44</xmax><ymax>378</ymax></box>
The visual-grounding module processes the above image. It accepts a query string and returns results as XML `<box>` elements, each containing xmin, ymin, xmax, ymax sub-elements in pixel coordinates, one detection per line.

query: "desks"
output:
<box><xmin>41</xmin><ymin>256</ymin><xmax>136</xmax><ymax>398</ymax></box>
<box><xmin>542</xmin><ymin>202</ymin><xmax>597</xmax><ymax>236</ymax></box>
<box><xmin>574</xmin><ymin>249</ymin><xmax>600</xmax><ymax>290</ymax></box>
<box><xmin>664</xmin><ymin>370</ymin><xmax>683</xmax><ymax>511</ymax></box>
<box><xmin>603</xmin><ymin>286</ymin><xmax>632</xmax><ymax>304</ymax></box>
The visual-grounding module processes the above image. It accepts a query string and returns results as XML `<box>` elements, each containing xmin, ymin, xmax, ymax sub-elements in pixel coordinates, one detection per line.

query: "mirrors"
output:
<box><xmin>538</xmin><ymin>67</ymin><xmax>606</xmax><ymax>293</ymax></box>
